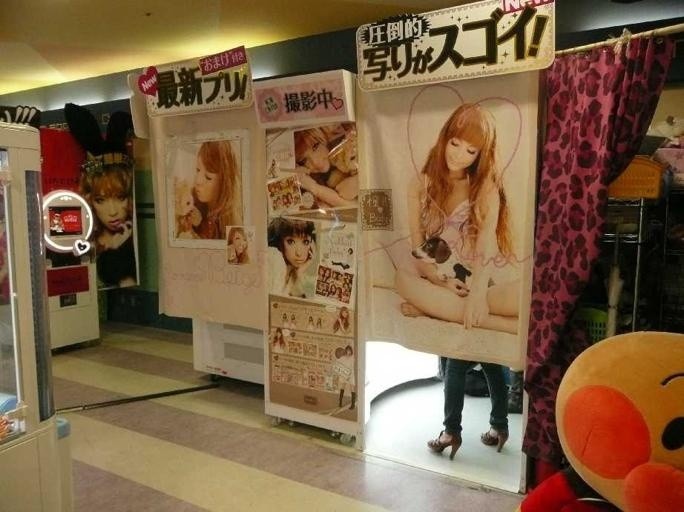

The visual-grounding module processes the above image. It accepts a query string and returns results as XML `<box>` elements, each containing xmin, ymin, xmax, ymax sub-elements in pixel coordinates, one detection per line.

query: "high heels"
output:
<box><xmin>427</xmin><ymin>430</ymin><xmax>462</xmax><ymax>460</ymax></box>
<box><xmin>481</xmin><ymin>424</ymin><xmax>509</xmax><ymax>453</ymax></box>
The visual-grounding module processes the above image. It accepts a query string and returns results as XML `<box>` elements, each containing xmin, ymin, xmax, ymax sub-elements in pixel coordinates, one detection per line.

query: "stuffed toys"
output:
<box><xmin>551</xmin><ymin>329</ymin><xmax>683</xmax><ymax>512</ymax></box>
<box><xmin>325</xmin><ymin>121</ymin><xmax>361</xmax><ymax>201</ymax></box>
<box><xmin>226</xmin><ymin>244</ymin><xmax>237</xmax><ymax>264</ymax></box>
<box><xmin>172</xmin><ymin>180</ymin><xmax>202</xmax><ymax>240</ymax></box>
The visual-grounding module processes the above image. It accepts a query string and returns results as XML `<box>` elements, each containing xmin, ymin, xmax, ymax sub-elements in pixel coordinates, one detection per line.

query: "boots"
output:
<box><xmin>338</xmin><ymin>389</ymin><xmax>356</xmax><ymax>410</ymax></box>
<box><xmin>507</xmin><ymin>369</ymin><xmax>523</xmax><ymax>414</ymax></box>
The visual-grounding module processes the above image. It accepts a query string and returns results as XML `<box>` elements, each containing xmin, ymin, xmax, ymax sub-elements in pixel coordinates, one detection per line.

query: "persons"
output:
<box><xmin>266</xmin><ymin>159</ymin><xmax>279</xmax><ymax>179</ymax></box>
<box><xmin>305</xmin><ymin>315</ymin><xmax>315</xmax><ymax>331</ymax></box>
<box><xmin>425</xmin><ymin>356</ymin><xmax>512</xmax><ymax>463</ymax></box>
<box><xmin>264</xmin><ymin>218</ymin><xmax>318</xmax><ymax>300</ymax></box>
<box><xmin>279</xmin><ymin>312</ymin><xmax>289</xmax><ymax>330</ymax></box>
<box><xmin>293</xmin><ymin>121</ymin><xmax>360</xmax><ymax>212</ymax></box>
<box><xmin>315</xmin><ymin>318</ymin><xmax>321</xmax><ymax>329</ymax></box>
<box><xmin>389</xmin><ymin>101</ymin><xmax>525</xmax><ymax>338</ymax></box>
<box><xmin>266</xmin><ymin>174</ymin><xmax>301</xmax><ymax>213</ymax></box>
<box><xmin>313</xmin><ymin>265</ymin><xmax>354</xmax><ymax>304</ymax></box>
<box><xmin>330</xmin><ymin>247</ymin><xmax>354</xmax><ymax>269</ymax></box>
<box><xmin>48</xmin><ymin>214</ymin><xmax>63</xmax><ymax>235</ymax></box>
<box><xmin>75</xmin><ymin>151</ymin><xmax>138</xmax><ymax>293</ymax></box>
<box><xmin>190</xmin><ymin>141</ymin><xmax>240</xmax><ymax>242</ymax></box>
<box><xmin>332</xmin><ymin>305</ymin><xmax>351</xmax><ymax>334</ymax></box>
<box><xmin>332</xmin><ymin>344</ymin><xmax>355</xmax><ymax>410</ymax></box>
<box><xmin>288</xmin><ymin>313</ymin><xmax>296</xmax><ymax>330</ymax></box>
<box><xmin>228</xmin><ymin>227</ymin><xmax>251</xmax><ymax>265</ymax></box>
<box><xmin>269</xmin><ymin>327</ymin><xmax>286</xmax><ymax>356</ymax></box>
